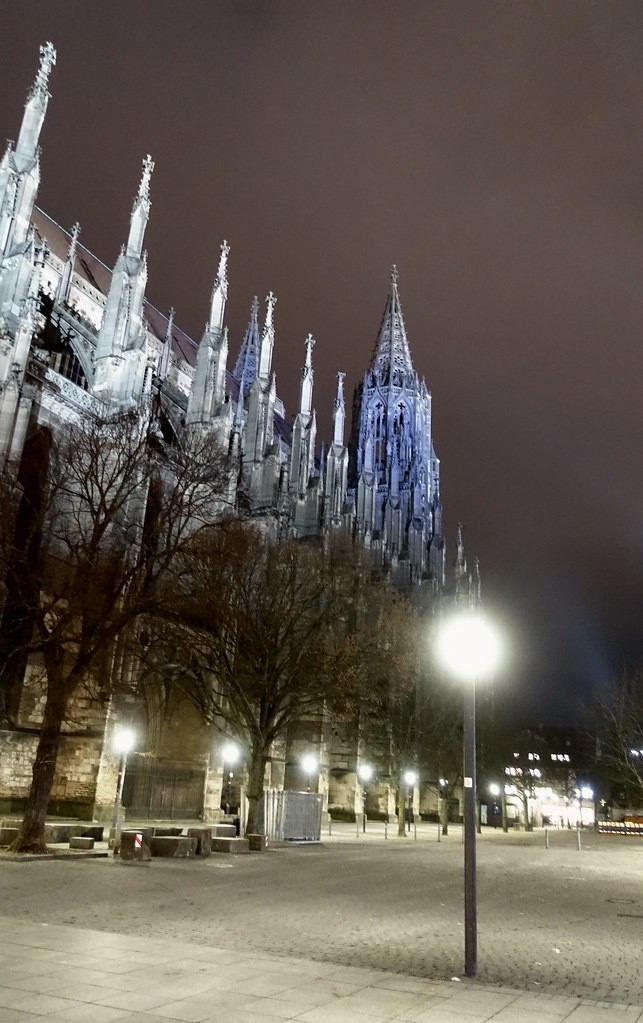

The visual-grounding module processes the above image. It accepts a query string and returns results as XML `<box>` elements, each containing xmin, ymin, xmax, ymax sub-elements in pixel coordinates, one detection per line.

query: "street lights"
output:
<box><xmin>490</xmin><ymin>782</ymin><xmax>499</xmax><ymax>829</ymax></box>
<box><xmin>406</xmin><ymin>771</ymin><xmax>414</xmax><ymax>830</ymax></box>
<box><xmin>110</xmin><ymin>722</ymin><xmax>136</xmax><ymax>839</ymax></box>
<box><xmin>423</xmin><ymin>605</ymin><xmax>514</xmax><ymax>977</ymax></box>
<box><xmin>221</xmin><ymin>740</ymin><xmax>238</xmax><ymax>820</ymax></box>
<box><xmin>302</xmin><ymin>753</ymin><xmax>317</xmax><ymax>793</ymax></box>
<box><xmin>358</xmin><ymin>763</ymin><xmax>372</xmax><ymax>832</ymax></box>
